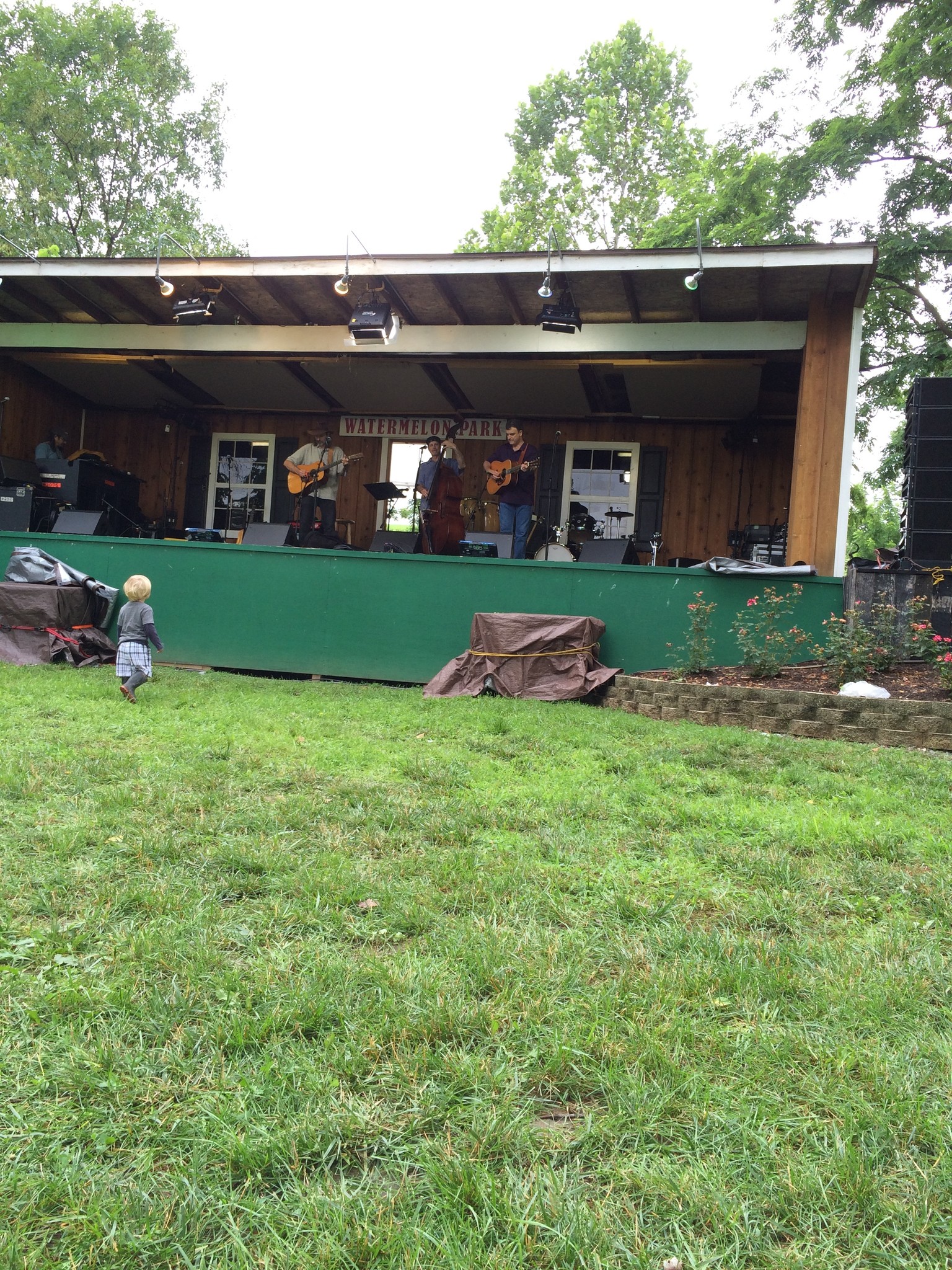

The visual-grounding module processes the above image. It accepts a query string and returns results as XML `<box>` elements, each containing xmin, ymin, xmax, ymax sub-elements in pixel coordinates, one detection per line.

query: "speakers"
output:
<box><xmin>753</xmin><ymin>544</ymin><xmax>787</xmax><ymax>566</ymax></box>
<box><xmin>51</xmin><ymin>509</ymin><xmax>114</xmax><ymax>536</ymax></box>
<box><xmin>369</xmin><ymin>530</ymin><xmax>423</xmax><ymax>554</ymax></box>
<box><xmin>898</xmin><ymin>375</ymin><xmax>952</xmax><ymax>574</ymax></box>
<box><xmin>466</xmin><ymin>530</ymin><xmax>515</xmax><ymax>559</ymax></box>
<box><xmin>0</xmin><ymin>455</ymin><xmax>42</xmax><ymax>487</ymax></box>
<box><xmin>578</xmin><ymin>538</ymin><xmax>640</xmax><ymax>565</ymax></box>
<box><xmin>241</xmin><ymin>522</ymin><xmax>298</xmax><ymax>547</ymax></box>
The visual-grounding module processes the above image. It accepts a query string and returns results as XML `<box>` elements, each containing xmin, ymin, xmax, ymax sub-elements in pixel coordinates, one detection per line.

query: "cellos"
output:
<box><xmin>422</xmin><ymin>414</ymin><xmax>464</xmax><ymax>554</ymax></box>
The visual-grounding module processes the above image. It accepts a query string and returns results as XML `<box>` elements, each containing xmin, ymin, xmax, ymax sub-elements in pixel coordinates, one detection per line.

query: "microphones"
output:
<box><xmin>227</xmin><ymin>455</ymin><xmax>231</xmax><ymax>462</ymax></box>
<box><xmin>326</xmin><ymin>437</ymin><xmax>331</xmax><ymax>442</ymax></box>
<box><xmin>420</xmin><ymin>446</ymin><xmax>427</xmax><ymax>449</ymax></box>
<box><xmin>555</xmin><ymin>431</ymin><xmax>561</xmax><ymax>436</ymax></box>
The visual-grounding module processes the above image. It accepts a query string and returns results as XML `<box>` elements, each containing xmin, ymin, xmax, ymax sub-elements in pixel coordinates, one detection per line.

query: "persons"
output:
<box><xmin>482</xmin><ymin>418</ymin><xmax>539</xmax><ymax>560</ymax></box>
<box><xmin>416</xmin><ymin>435</ymin><xmax>467</xmax><ymax>549</ymax></box>
<box><xmin>117</xmin><ymin>575</ymin><xmax>164</xmax><ymax>703</ymax></box>
<box><xmin>284</xmin><ymin>422</ymin><xmax>350</xmax><ymax>550</ymax></box>
<box><xmin>34</xmin><ymin>428</ymin><xmax>71</xmax><ymax>466</ymax></box>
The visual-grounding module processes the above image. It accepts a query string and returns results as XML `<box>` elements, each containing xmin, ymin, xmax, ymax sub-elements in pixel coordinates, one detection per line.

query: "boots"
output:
<box><xmin>120</xmin><ymin>669</ymin><xmax>148</xmax><ymax>704</ymax></box>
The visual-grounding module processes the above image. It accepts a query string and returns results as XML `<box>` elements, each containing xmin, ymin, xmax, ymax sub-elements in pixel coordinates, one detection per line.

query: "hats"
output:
<box><xmin>426</xmin><ymin>436</ymin><xmax>440</xmax><ymax>444</ymax></box>
<box><xmin>307</xmin><ymin>421</ymin><xmax>334</xmax><ymax>436</ymax></box>
<box><xmin>53</xmin><ymin>426</ymin><xmax>70</xmax><ymax>441</ymax></box>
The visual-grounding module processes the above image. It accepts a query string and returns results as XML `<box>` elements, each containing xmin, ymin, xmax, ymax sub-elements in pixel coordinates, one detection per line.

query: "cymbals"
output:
<box><xmin>604</xmin><ymin>511</ymin><xmax>634</xmax><ymax>518</ymax></box>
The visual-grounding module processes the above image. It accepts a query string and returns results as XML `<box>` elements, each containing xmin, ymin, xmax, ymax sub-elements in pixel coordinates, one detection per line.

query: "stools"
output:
<box><xmin>335</xmin><ymin>518</ymin><xmax>355</xmax><ymax>545</ymax></box>
<box><xmin>37</xmin><ymin>496</ymin><xmax>58</xmax><ymax>533</ymax></box>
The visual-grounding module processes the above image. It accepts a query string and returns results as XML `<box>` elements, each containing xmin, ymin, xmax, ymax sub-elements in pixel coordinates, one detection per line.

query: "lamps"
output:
<box><xmin>534</xmin><ymin>303</ymin><xmax>584</xmax><ymax>335</ymax></box>
<box><xmin>536</xmin><ymin>224</ymin><xmax>564</xmax><ymax>299</ymax></box>
<box><xmin>684</xmin><ymin>218</ymin><xmax>703</xmax><ymax>291</ymax></box>
<box><xmin>152</xmin><ymin>233</ymin><xmax>200</xmax><ymax>297</ymax></box>
<box><xmin>168</xmin><ymin>295</ymin><xmax>214</xmax><ymax>325</ymax></box>
<box><xmin>334</xmin><ymin>230</ymin><xmax>376</xmax><ymax>296</ymax></box>
<box><xmin>351</xmin><ymin>303</ymin><xmax>401</xmax><ymax>346</ymax></box>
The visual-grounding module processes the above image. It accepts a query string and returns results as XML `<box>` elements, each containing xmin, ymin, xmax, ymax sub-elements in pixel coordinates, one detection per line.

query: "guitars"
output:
<box><xmin>287</xmin><ymin>452</ymin><xmax>364</xmax><ymax>498</ymax></box>
<box><xmin>486</xmin><ymin>457</ymin><xmax>540</xmax><ymax>495</ymax></box>
<box><xmin>235</xmin><ymin>498</ymin><xmax>258</xmax><ymax>544</ymax></box>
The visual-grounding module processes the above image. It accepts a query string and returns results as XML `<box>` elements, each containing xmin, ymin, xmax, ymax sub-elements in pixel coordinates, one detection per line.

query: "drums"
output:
<box><xmin>533</xmin><ymin>543</ymin><xmax>574</xmax><ymax>561</ymax></box>
<box><xmin>567</xmin><ymin>514</ymin><xmax>597</xmax><ymax>544</ymax></box>
<box><xmin>481</xmin><ymin>500</ymin><xmax>500</xmax><ymax>532</ymax></box>
<box><xmin>459</xmin><ymin>496</ymin><xmax>483</xmax><ymax>531</ymax></box>
